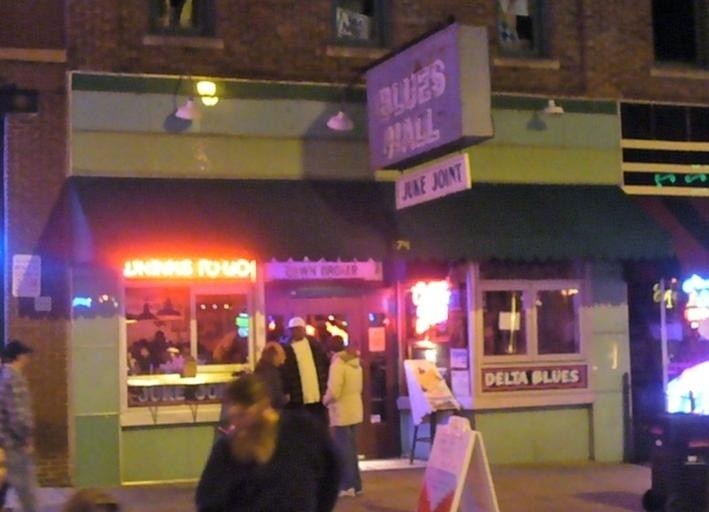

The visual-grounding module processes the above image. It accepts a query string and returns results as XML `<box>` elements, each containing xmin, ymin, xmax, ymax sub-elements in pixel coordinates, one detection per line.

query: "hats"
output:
<box><xmin>286</xmin><ymin>317</ymin><xmax>306</xmax><ymax>330</ymax></box>
<box><xmin>5</xmin><ymin>341</ymin><xmax>34</xmax><ymax>358</ymax></box>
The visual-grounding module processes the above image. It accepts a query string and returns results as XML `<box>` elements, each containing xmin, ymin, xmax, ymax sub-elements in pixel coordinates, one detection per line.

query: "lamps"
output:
<box><xmin>326</xmin><ymin>84</ymin><xmax>353</xmax><ymax>133</ymax></box>
<box><xmin>173</xmin><ymin>72</ymin><xmax>204</xmax><ymax>122</ymax></box>
<box><xmin>124</xmin><ymin>288</ymin><xmax>183</xmax><ymax>324</ymax></box>
<box><xmin>539</xmin><ymin>92</ymin><xmax>565</xmax><ymax>118</ymax></box>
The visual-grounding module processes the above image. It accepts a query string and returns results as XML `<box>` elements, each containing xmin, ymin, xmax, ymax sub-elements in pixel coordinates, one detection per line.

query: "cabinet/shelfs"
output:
<box><xmin>404</xmin><ymin>360</ymin><xmax>465</xmax><ymax>464</ymax></box>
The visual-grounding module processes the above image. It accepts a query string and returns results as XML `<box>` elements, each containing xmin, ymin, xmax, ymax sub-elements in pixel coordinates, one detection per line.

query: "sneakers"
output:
<box><xmin>338</xmin><ymin>487</ymin><xmax>362</xmax><ymax>498</ymax></box>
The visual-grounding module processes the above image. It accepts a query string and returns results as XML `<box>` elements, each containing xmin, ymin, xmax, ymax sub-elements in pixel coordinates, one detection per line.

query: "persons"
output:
<box><xmin>196</xmin><ymin>318</ymin><xmax>364</xmax><ymax>512</ymax></box>
<box><xmin>125</xmin><ymin>324</ymin><xmax>241</xmax><ymax>402</ymax></box>
<box><xmin>1</xmin><ymin>339</ymin><xmax>45</xmax><ymax>511</ymax></box>
